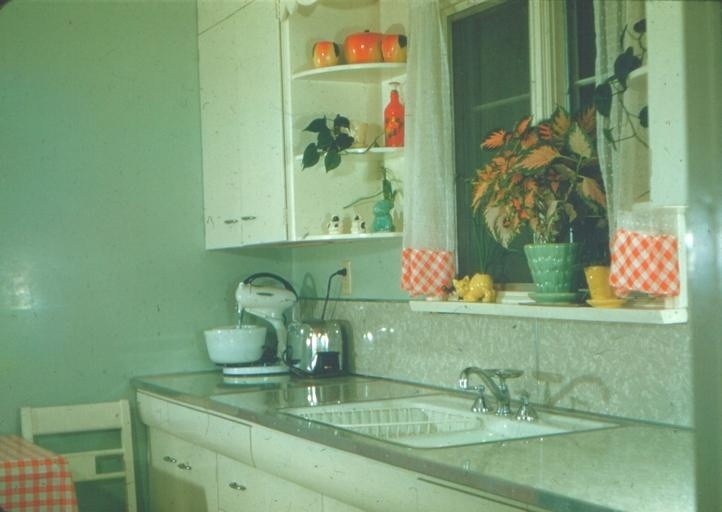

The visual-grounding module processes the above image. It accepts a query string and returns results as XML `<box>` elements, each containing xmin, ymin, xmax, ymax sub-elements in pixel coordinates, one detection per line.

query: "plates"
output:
<box><xmin>528</xmin><ymin>289</ymin><xmax>588</xmax><ymax>306</ymax></box>
<box><xmin>582</xmin><ymin>297</ymin><xmax>627</xmax><ymax>308</ymax></box>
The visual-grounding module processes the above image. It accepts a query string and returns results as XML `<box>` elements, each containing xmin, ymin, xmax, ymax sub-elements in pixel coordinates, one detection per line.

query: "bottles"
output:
<box><xmin>382</xmin><ymin>88</ymin><xmax>406</xmax><ymax>146</ymax></box>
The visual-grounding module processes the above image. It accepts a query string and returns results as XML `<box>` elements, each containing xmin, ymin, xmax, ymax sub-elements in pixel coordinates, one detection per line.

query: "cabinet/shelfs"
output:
<box><xmin>415</xmin><ymin>477</ymin><xmax>532</xmax><ymax>512</ymax></box>
<box><xmin>293</xmin><ymin>61</ymin><xmax>416</xmax><ymax>242</ymax></box>
<box><xmin>146</xmin><ymin>427</ymin><xmax>218</xmax><ymax>512</ymax></box>
<box><xmin>221</xmin><ymin>457</ymin><xmax>367</xmax><ymax>512</ymax></box>
<box><xmin>196</xmin><ymin>0</ymin><xmax>288</xmax><ymax>251</ymax></box>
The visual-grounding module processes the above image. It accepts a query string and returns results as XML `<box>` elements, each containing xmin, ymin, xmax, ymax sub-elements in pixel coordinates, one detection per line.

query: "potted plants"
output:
<box><xmin>588</xmin><ymin>15</ymin><xmax>651</xmax><ymax>199</ymax></box>
<box><xmin>584</xmin><ymin>213</ymin><xmax>623</xmax><ymax>309</ymax></box>
<box><xmin>452</xmin><ymin>212</ymin><xmax>522</xmax><ymax>304</ymax></box>
<box><xmin>470</xmin><ymin>104</ymin><xmax>610</xmax><ymax>304</ymax></box>
<box><xmin>301</xmin><ymin>107</ymin><xmax>383</xmax><ymax>172</ymax></box>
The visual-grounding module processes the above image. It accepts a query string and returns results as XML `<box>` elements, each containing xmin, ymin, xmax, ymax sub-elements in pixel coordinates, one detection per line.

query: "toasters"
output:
<box><xmin>284</xmin><ymin>319</ymin><xmax>345</xmax><ymax>382</ymax></box>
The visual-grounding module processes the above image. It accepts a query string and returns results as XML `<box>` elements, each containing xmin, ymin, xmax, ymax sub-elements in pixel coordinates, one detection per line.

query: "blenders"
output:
<box><xmin>200</xmin><ymin>271</ymin><xmax>300</xmax><ymax>378</ymax></box>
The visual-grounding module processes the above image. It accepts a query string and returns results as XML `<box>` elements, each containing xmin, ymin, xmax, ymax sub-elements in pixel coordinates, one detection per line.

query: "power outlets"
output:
<box><xmin>339</xmin><ymin>262</ymin><xmax>349</xmax><ymax>295</ymax></box>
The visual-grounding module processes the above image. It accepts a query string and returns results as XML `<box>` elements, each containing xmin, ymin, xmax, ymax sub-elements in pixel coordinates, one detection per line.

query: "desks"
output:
<box><xmin>0</xmin><ymin>432</ymin><xmax>79</xmax><ymax>512</ymax></box>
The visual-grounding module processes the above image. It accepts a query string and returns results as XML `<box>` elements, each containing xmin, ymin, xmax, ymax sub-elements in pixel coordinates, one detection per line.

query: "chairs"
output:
<box><xmin>21</xmin><ymin>398</ymin><xmax>140</xmax><ymax>511</ymax></box>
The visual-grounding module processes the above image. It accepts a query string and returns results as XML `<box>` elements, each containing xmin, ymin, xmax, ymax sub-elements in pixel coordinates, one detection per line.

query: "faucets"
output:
<box><xmin>458</xmin><ymin>365</ymin><xmax>523</xmax><ymax>416</ymax></box>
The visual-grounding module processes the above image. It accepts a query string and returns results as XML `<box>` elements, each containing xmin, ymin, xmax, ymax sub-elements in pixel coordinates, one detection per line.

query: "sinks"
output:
<box><xmin>278</xmin><ymin>394</ymin><xmax>624</xmax><ymax>449</ymax></box>
